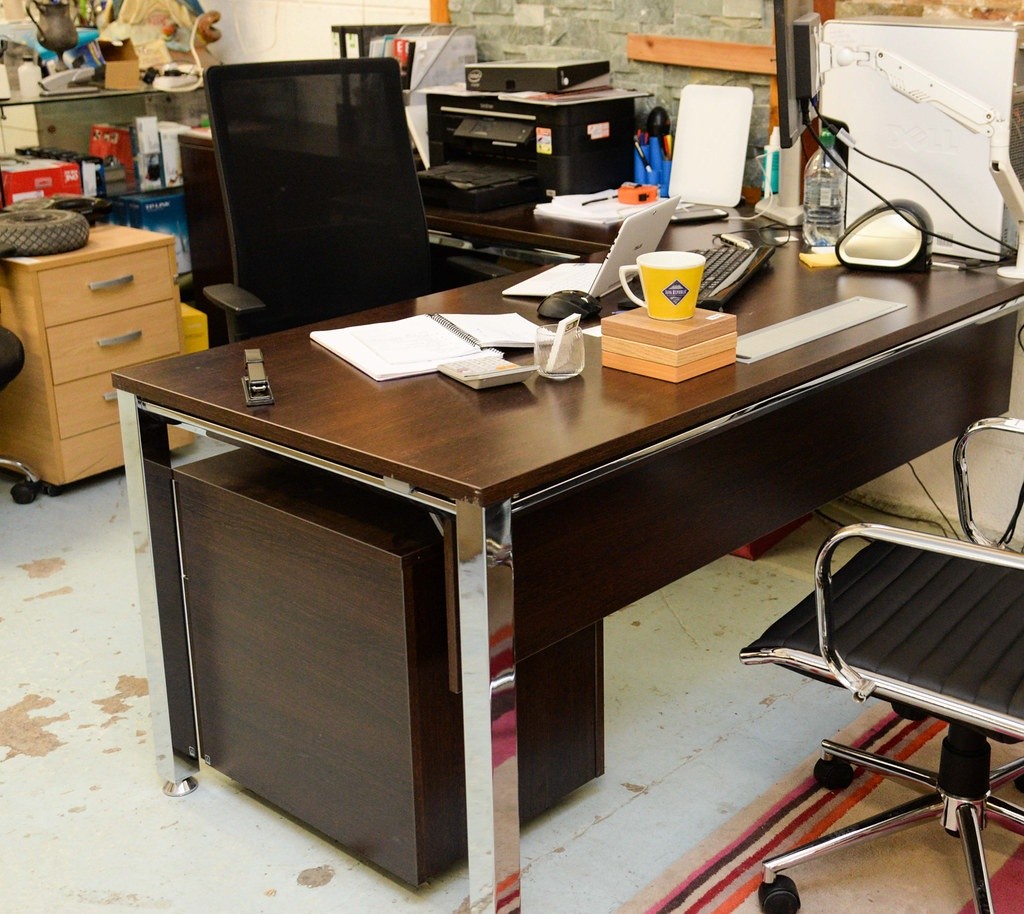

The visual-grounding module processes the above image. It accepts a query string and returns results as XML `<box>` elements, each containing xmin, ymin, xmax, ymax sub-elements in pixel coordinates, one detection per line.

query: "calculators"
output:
<box><xmin>437</xmin><ymin>356</ymin><xmax>538</xmax><ymax>390</ymax></box>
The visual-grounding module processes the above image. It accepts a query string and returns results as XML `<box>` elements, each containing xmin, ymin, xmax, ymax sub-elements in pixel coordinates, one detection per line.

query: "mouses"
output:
<box><xmin>537</xmin><ymin>290</ymin><xmax>603</xmax><ymax>320</ymax></box>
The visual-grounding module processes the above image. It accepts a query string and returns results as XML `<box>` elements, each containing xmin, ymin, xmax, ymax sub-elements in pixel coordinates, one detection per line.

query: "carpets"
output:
<box><xmin>603</xmin><ymin>701</ymin><xmax>1024</xmax><ymax>914</ymax></box>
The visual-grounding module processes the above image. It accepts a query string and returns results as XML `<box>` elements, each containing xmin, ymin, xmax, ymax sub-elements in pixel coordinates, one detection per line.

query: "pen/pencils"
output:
<box><xmin>662</xmin><ymin>134</ymin><xmax>672</xmax><ymax>160</ymax></box>
<box><xmin>633</xmin><ymin>137</ymin><xmax>652</xmax><ymax>171</ymax></box>
<box><xmin>634</xmin><ymin>129</ymin><xmax>649</xmax><ymax>144</ymax></box>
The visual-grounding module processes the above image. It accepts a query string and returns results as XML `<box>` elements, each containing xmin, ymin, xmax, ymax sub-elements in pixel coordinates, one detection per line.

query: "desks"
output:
<box><xmin>0</xmin><ymin>86</ymin><xmax>207</xmax><ymax>157</ymax></box>
<box><xmin>109</xmin><ymin>126</ymin><xmax>1024</xmax><ymax>889</ymax></box>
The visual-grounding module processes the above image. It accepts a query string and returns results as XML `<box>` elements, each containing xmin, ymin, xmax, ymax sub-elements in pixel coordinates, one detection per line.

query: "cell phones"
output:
<box><xmin>669</xmin><ymin>209</ymin><xmax>729</xmax><ymax>223</ymax></box>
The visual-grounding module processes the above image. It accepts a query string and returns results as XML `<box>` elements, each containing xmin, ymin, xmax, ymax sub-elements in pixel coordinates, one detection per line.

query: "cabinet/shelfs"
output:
<box><xmin>0</xmin><ymin>222</ymin><xmax>196</xmax><ymax>498</ymax></box>
<box><xmin>171</xmin><ymin>447</ymin><xmax>606</xmax><ymax>887</ymax></box>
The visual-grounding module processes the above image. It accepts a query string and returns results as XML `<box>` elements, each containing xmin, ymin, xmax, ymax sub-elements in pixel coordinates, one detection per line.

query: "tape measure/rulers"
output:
<box><xmin>617</xmin><ymin>181</ymin><xmax>658</xmax><ymax>205</ymax></box>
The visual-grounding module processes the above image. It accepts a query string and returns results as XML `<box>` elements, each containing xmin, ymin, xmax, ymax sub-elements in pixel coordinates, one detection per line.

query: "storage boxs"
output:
<box><xmin>601</xmin><ymin>307</ymin><xmax>738</xmax><ymax>384</ymax></box>
<box><xmin>464</xmin><ymin>58</ymin><xmax>611</xmax><ymax>93</ymax></box>
<box><xmin>0</xmin><ymin>154</ymin><xmax>83</xmax><ymax>208</ymax></box>
<box><xmin>90</xmin><ymin>117</ymin><xmax>193</xmax><ymax>277</ymax></box>
<box><xmin>97</xmin><ymin>39</ymin><xmax>140</xmax><ymax>90</ymax></box>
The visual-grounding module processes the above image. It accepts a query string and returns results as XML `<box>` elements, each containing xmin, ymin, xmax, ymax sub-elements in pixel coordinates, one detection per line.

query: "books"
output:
<box><xmin>308</xmin><ymin>312</ymin><xmax>556</xmax><ymax>381</ymax></box>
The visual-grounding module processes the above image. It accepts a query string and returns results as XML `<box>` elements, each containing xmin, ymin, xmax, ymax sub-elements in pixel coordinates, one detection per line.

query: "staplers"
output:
<box><xmin>241</xmin><ymin>349</ymin><xmax>274</xmax><ymax>406</ymax></box>
<box><xmin>38</xmin><ymin>66</ymin><xmax>99</xmax><ymax>96</ymax></box>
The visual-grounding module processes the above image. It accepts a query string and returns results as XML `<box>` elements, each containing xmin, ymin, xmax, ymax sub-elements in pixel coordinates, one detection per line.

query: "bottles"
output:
<box><xmin>802</xmin><ymin>135</ymin><xmax>847</xmax><ymax>245</ymax></box>
<box><xmin>18</xmin><ymin>56</ymin><xmax>42</xmax><ymax>99</ymax></box>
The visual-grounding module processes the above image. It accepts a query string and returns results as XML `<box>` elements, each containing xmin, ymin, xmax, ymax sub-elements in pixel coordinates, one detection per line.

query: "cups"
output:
<box><xmin>619</xmin><ymin>251</ymin><xmax>706</xmax><ymax>319</ymax></box>
<box><xmin>533</xmin><ymin>324</ymin><xmax>586</xmax><ymax>379</ymax></box>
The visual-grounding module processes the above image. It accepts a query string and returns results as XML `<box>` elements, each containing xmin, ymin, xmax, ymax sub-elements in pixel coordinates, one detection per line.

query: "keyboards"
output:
<box><xmin>617</xmin><ymin>244</ymin><xmax>778</xmax><ymax>311</ymax></box>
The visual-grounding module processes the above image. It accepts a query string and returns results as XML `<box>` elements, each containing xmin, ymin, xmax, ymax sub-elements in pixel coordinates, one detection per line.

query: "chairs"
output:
<box><xmin>739</xmin><ymin>417</ymin><xmax>1024</xmax><ymax>914</ymax></box>
<box><xmin>0</xmin><ymin>244</ymin><xmax>40</xmax><ymax>506</ymax></box>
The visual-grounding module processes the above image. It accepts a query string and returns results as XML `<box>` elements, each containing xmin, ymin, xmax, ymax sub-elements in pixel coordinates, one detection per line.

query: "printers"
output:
<box><xmin>416</xmin><ymin>93</ymin><xmax>637</xmax><ymax>213</ymax></box>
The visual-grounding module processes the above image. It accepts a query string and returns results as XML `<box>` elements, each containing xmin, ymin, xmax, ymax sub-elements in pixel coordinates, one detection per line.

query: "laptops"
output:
<box><xmin>502</xmin><ymin>195</ymin><xmax>682</xmax><ymax>300</ymax></box>
